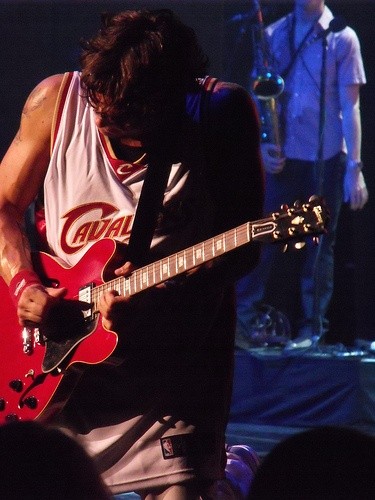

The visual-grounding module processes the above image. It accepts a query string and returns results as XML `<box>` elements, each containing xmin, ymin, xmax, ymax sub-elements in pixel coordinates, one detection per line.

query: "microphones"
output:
<box><xmin>314</xmin><ymin>16</ymin><xmax>348</xmax><ymax>40</ymax></box>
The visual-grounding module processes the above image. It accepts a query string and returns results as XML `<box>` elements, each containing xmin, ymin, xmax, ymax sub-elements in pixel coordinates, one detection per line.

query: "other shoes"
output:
<box><xmin>282</xmin><ymin>325</ymin><xmax>327</xmax><ymax>352</ymax></box>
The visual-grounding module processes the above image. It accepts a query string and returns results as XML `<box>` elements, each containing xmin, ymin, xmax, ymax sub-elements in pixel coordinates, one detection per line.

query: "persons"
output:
<box><xmin>0</xmin><ymin>6</ymin><xmax>267</xmax><ymax>500</ymax></box>
<box><xmin>235</xmin><ymin>0</ymin><xmax>368</xmax><ymax>354</ymax></box>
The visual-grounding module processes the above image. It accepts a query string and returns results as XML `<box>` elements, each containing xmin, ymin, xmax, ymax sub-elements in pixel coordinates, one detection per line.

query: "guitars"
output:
<box><xmin>0</xmin><ymin>192</ymin><xmax>330</xmax><ymax>429</ymax></box>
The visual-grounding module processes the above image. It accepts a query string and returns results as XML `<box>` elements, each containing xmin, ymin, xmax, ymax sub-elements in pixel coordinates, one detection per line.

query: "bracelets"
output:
<box><xmin>346</xmin><ymin>160</ymin><xmax>361</xmax><ymax>170</ymax></box>
<box><xmin>9</xmin><ymin>271</ymin><xmax>42</xmax><ymax>307</ymax></box>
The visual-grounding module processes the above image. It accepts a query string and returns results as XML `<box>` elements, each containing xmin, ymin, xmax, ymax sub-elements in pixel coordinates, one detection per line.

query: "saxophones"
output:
<box><xmin>250</xmin><ymin>0</ymin><xmax>286</xmax><ymax>175</ymax></box>
